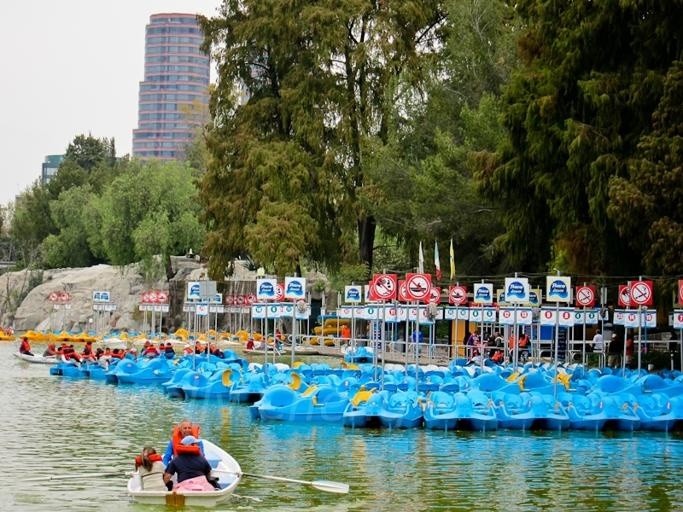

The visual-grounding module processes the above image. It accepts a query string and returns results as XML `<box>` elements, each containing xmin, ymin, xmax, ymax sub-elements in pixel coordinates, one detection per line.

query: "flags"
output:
<box><xmin>448</xmin><ymin>239</ymin><xmax>455</xmax><ymax>284</ymax></box>
<box><xmin>418</xmin><ymin>240</ymin><xmax>425</xmax><ymax>275</ymax></box>
<box><xmin>431</xmin><ymin>240</ymin><xmax>443</xmax><ymax>281</ymax></box>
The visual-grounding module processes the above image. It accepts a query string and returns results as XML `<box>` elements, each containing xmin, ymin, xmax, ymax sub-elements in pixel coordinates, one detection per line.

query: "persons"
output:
<box><xmin>340</xmin><ymin>323</ymin><xmax>350</xmax><ymax>346</ymax></box>
<box><xmin>135</xmin><ymin>445</ymin><xmax>168</xmax><ymax>490</ymax></box>
<box><xmin>160</xmin><ymin>436</ymin><xmax>222</xmax><ymax>492</ymax></box>
<box><xmin>410</xmin><ymin>328</ymin><xmax>422</xmax><ymax>358</ymax></box>
<box><xmin>17</xmin><ymin>328</ymin><xmax>286</xmax><ymax>364</ymax></box>
<box><xmin>463</xmin><ymin>327</ymin><xmax>636</xmax><ymax>369</ymax></box>
<box><xmin>161</xmin><ymin>421</ymin><xmax>204</xmax><ymax>465</ymax></box>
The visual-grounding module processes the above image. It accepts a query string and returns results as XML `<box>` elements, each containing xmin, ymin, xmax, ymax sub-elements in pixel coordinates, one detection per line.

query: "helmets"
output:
<box><xmin>181</xmin><ymin>435</ymin><xmax>200</xmax><ymax>446</ymax></box>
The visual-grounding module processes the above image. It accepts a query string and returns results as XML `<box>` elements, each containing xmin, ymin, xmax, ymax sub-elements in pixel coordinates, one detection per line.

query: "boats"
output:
<box><xmin>242</xmin><ymin>347</ymin><xmax>285</xmax><ymax>355</ymax></box>
<box><xmin>343</xmin><ymin>346</ymin><xmax>378</xmax><ymax>364</ymax></box>
<box><xmin>126</xmin><ymin>439</ymin><xmax>244</xmax><ymax>498</ymax></box>
<box><xmin>44</xmin><ymin>348</ymin><xmax>683</xmax><ymax>432</ymax></box>
<box><xmin>10</xmin><ymin>349</ymin><xmax>61</xmax><ymax>363</ymax></box>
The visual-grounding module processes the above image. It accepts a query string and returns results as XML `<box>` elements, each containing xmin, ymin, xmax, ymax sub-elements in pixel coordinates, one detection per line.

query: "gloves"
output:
<box><xmin>166</xmin><ymin>481</ymin><xmax>173</xmax><ymax>491</ymax></box>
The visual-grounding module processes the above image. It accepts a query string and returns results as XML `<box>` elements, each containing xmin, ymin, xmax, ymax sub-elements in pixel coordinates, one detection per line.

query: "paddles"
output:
<box><xmin>212</xmin><ymin>469</ymin><xmax>348</xmax><ymax>494</ymax></box>
<box><xmin>22</xmin><ymin>472</ymin><xmax>139</xmax><ymax>481</ymax></box>
